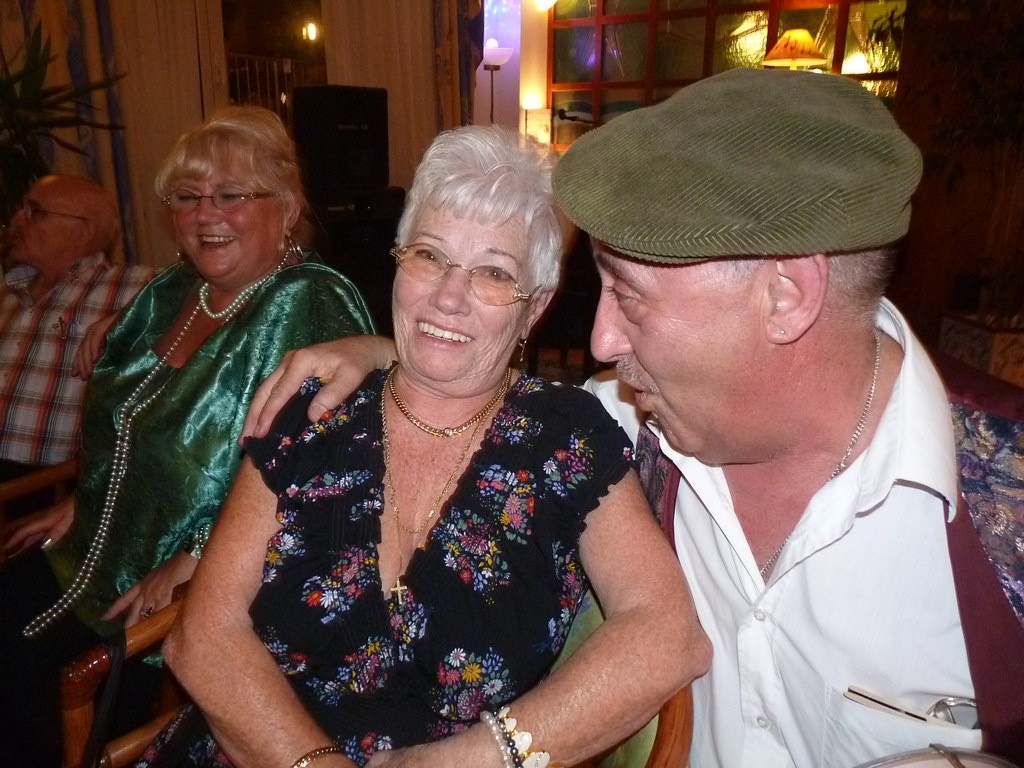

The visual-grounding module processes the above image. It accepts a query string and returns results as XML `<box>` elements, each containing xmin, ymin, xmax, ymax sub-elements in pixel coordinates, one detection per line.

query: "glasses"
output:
<box><xmin>389</xmin><ymin>243</ymin><xmax>541</xmax><ymax>306</ymax></box>
<box><xmin>163</xmin><ymin>189</ymin><xmax>281</xmax><ymax>214</ymax></box>
<box><xmin>20</xmin><ymin>193</ymin><xmax>88</xmax><ymax>221</ymax></box>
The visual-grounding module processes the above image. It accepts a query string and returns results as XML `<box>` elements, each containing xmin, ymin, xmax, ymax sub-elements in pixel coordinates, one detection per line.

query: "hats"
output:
<box><xmin>551</xmin><ymin>70</ymin><xmax>922</xmax><ymax>264</ymax></box>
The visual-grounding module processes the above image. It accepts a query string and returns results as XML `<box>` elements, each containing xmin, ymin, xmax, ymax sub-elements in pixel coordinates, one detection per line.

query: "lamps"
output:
<box><xmin>484</xmin><ymin>39</ymin><xmax>514</xmax><ymax>124</ymax></box>
<box><xmin>762</xmin><ymin>28</ymin><xmax>827</xmax><ymax>70</ymax></box>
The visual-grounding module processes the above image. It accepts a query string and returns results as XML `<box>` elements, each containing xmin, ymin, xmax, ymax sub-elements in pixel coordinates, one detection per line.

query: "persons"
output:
<box><xmin>0</xmin><ymin>104</ymin><xmax>375</xmax><ymax>747</ymax></box>
<box><xmin>0</xmin><ymin>171</ymin><xmax>171</xmax><ymax>524</ymax></box>
<box><xmin>128</xmin><ymin>124</ymin><xmax>714</xmax><ymax>768</ymax></box>
<box><xmin>238</xmin><ymin>69</ymin><xmax>1024</xmax><ymax>768</ymax></box>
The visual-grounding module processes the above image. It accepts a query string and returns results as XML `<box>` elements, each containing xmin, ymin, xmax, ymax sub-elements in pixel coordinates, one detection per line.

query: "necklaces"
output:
<box><xmin>21</xmin><ymin>249</ymin><xmax>294</xmax><ymax>637</ymax></box>
<box><xmin>759</xmin><ymin>328</ymin><xmax>883</xmax><ymax>577</ymax></box>
<box><xmin>381</xmin><ymin>364</ymin><xmax>512</xmax><ymax>604</ymax></box>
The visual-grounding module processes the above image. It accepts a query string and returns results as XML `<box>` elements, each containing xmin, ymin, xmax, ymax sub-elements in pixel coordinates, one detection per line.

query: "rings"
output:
<box><xmin>140</xmin><ymin>605</ymin><xmax>155</xmax><ymax>617</ymax></box>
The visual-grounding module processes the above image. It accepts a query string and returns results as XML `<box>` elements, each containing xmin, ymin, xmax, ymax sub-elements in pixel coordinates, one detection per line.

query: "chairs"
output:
<box><xmin>1</xmin><ymin>254</ymin><xmax>696</xmax><ymax>768</ymax></box>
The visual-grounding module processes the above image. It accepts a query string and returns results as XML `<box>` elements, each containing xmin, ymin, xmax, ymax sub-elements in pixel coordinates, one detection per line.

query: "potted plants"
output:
<box><xmin>867</xmin><ymin>0</ymin><xmax>1024</xmax><ymax>386</ymax></box>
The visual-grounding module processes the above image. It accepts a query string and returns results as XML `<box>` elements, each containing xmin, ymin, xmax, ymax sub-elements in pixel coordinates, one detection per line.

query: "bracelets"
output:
<box><xmin>291</xmin><ymin>745</ymin><xmax>343</xmax><ymax>768</ymax></box>
<box><xmin>479</xmin><ymin>703</ymin><xmax>550</xmax><ymax>768</ymax></box>
